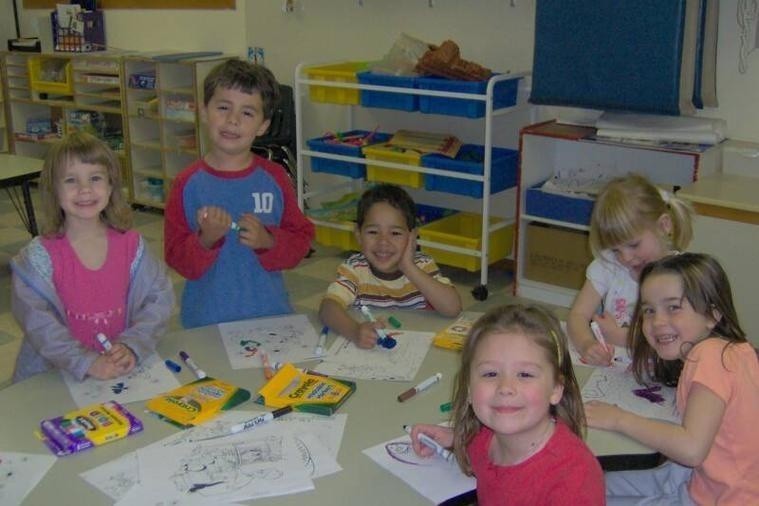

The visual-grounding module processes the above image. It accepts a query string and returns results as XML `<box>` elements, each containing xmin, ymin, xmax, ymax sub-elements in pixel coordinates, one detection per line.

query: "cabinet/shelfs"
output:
<box><xmin>510</xmin><ymin>117</ymin><xmax>728</xmax><ymax>312</ymax></box>
<box><xmin>671</xmin><ymin>173</ymin><xmax>759</xmax><ymax>352</ymax></box>
<box><xmin>1</xmin><ymin>45</ymin><xmax>14</xmax><ymax>156</ymax></box>
<box><xmin>121</xmin><ymin>51</ymin><xmax>242</xmax><ymax>213</ymax></box>
<box><xmin>292</xmin><ymin>62</ymin><xmax>536</xmax><ymax>299</ymax></box>
<box><xmin>10</xmin><ymin>47</ymin><xmax>141</xmax><ymax>209</ymax></box>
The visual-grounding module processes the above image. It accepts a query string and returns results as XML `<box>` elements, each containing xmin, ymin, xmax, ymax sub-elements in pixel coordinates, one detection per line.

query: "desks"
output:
<box><xmin>0</xmin><ymin>153</ymin><xmax>47</xmax><ymax>239</ymax></box>
<box><xmin>1</xmin><ymin>308</ymin><xmax>686</xmax><ymax>506</ymax></box>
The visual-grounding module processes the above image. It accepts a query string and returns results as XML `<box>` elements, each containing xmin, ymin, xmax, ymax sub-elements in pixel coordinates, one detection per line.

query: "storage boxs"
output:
<box><xmin>309</xmin><ymin>206</ymin><xmax>363</xmax><ymax>254</ymax></box>
<box><xmin>418</xmin><ymin>211</ymin><xmax>516</xmax><ymax>272</ymax></box>
<box><xmin>362</xmin><ymin>141</ymin><xmax>431</xmax><ymax>189</ymax></box>
<box><xmin>305</xmin><ymin>64</ymin><xmax>374</xmax><ymax>106</ymax></box>
<box><xmin>355</xmin><ymin>68</ymin><xmax>433</xmax><ymax>113</ymax></box>
<box><xmin>414</xmin><ymin>203</ymin><xmax>462</xmax><ymax>253</ymax></box>
<box><xmin>306</xmin><ymin>129</ymin><xmax>396</xmax><ymax>180</ymax></box>
<box><xmin>526</xmin><ymin>180</ymin><xmax>594</xmax><ymax>227</ymax></box>
<box><xmin>421</xmin><ymin>143</ymin><xmax>520</xmax><ymax>200</ymax></box>
<box><xmin>415</xmin><ymin>72</ymin><xmax>524</xmax><ymax>120</ymax></box>
<box><xmin>521</xmin><ymin>222</ymin><xmax>593</xmax><ymax>293</ymax></box>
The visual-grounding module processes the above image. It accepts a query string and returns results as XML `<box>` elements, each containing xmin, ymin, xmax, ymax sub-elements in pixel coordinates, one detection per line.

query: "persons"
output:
<box><xmin>10</xmin><ymin>134</ymin><xmax>176</xmax><ymax>384</ymax></box>
<box><xmin>566</xmin><ymin>172</ymin><xmax>694</xmax><ymax>367</ymax></box>
<box><xmin>410</xmin><ymin>301</ymin><xmax>606</xmax><ymax>505</ymax></box>
<box><xmin>164</xmin><ymin>59</ymin><xmax>316</xmax><ymax>328</ymax></box>
<box><xmin>318</xmin><ymin>185</ymin><xmax>462</xmax><ymax>349</ymax></box>
<box><xmin>581</xmin><ymin>254</ymin><xmax>758</xmax><ymax>506</ymax></box>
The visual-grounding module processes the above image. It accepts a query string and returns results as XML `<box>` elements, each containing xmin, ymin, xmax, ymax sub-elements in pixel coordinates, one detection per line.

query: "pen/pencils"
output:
<box><xmin>231</xmin><ymin>406</ymin><xmax>292</xmax><ymax>434</ymax></box>
<box><xmin>398</xmin><ymin>372</ymin><xmax>442</xmax><ymax>403</ymax></box>
<box><xmin>259</xmin><ymin>350</ymin><xmax>308</xmax><ymax>379</ymax></box>
<box><xmin>180</xmin><ymin>350</ymin><xmax>206</xmax><ymax>379</ymax></box>
<box><xmin>203</xmin><ymin>212</ymin><xmax>245</xmax><ymax>232</ymax></box>
<box><xmin>96</xmin><ymin>333</ymin><xmax>113</xmax><ymax>352</ymax></box>
<box><xmin>403</xmin><ymin>425</ymin><xmax>458</xmax><ymax>464</ymax></box>
<box><xmin>591</xmin><ymin>322</ymin><xmax>615</xmax><ymax>366</ymax></box>
<box><xmin>316</xmin><ymin>326</ymin><xmax>328</xmax><ymax>354</ymax></box>
<box><xmin>360</xmin><ymin>305</ymin><xmax>388</xmax><ymax>342</ymax></box>
